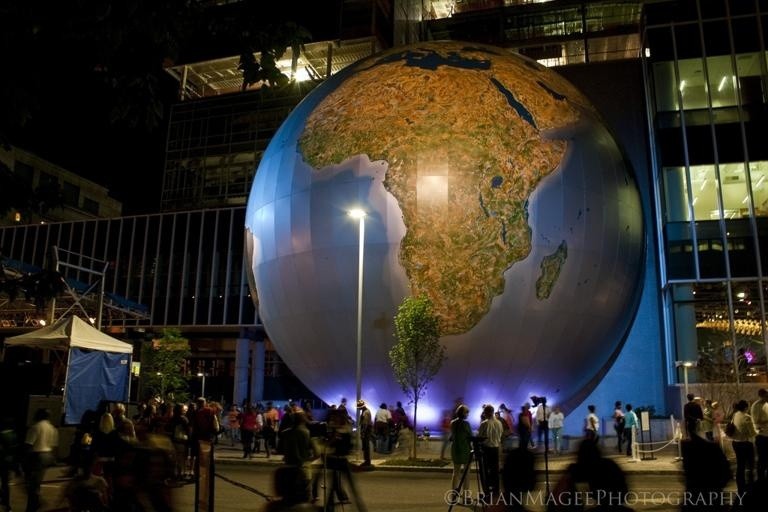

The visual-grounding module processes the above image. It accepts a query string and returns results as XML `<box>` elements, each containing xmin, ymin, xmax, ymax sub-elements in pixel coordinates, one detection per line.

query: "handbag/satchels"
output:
<box><xmin>725</xmin><ymin>422</ymin><xmax>734</xmax><ymax>436</ymax></box>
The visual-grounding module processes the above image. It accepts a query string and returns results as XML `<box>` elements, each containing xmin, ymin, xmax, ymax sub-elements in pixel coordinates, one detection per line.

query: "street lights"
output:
<box><xmin>196</xmin><ymin>372</ymin><xmax>207</xmax><ymax>397</ymax></box>
<box><xmin>678</xmin><ymin>359</ymin><xmax>696</xmax><ymax>398</ymax></box>
<box><xmin>343</xmin><ymin>205</ymin><xmax>368</xmax><ymax>426</ymax></box>
<box><xmin>155</xmin><ymin>372</ymin><xmax>165</xmax><ymax>400</ymax></box>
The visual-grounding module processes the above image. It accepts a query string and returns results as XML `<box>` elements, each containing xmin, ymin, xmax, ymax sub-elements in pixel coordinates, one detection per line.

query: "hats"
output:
<box><xmin>356</xmin><ymin>400</ymin><xmax>364</xmax><ymax>409</ymax></box>
